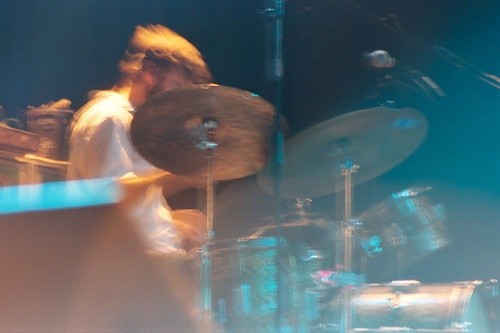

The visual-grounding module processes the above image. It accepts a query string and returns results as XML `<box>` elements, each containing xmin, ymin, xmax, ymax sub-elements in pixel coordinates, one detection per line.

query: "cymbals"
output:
<box><xmin>257</xmin><ymin>106</ymin><xmax>427</xmax><ymax>199</ymax></box>
<box><xmin>129</xmin><ymin>84</ymin><xmax>289</xmax><ymax>179</ymax></box>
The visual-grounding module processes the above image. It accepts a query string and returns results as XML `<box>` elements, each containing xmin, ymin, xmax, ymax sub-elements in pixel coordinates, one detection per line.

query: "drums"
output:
<box><xmin>331</xmin><ymin>280</ymin><xmax>482</xmax><ymax>332</ymax></box>
<box><xmin>354</xmin><ymin>185</ymin><xmax>455</xmax><ymax>272</ymax></box>
<box><xmin>185</xmin><ymin>235</ymin><xmax>297</xmax><ymax>333</ymax></box>
<box><xmin>255</xmin><ymin>218</ymin><xmax>365</xmax><ymax>310</ymax></box>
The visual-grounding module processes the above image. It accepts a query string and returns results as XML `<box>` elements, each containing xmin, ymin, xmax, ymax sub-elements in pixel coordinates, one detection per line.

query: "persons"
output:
<box><xmin>62</xmin><ymin>22</ymin><xmax>225</xmax><ymax>333</ymax></box>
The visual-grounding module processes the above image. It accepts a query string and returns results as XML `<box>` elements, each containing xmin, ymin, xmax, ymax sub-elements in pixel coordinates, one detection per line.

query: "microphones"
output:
<box><xmin>367</xmin><ymin>50</ymin><xmax>444</xmax><ymax>96</ymax></box>
